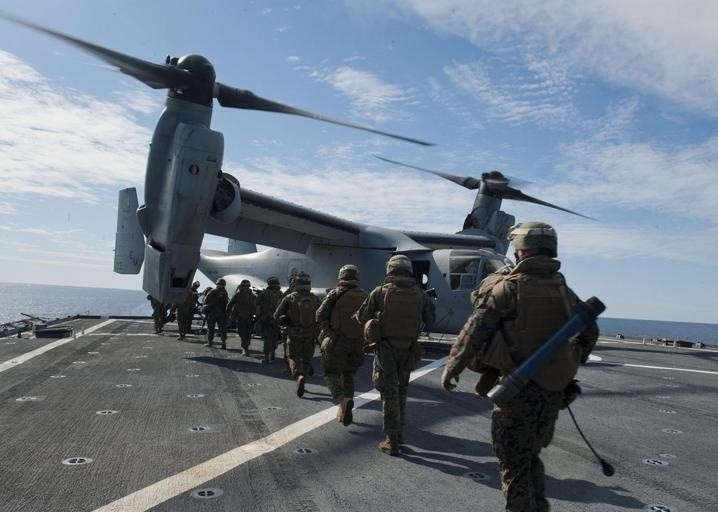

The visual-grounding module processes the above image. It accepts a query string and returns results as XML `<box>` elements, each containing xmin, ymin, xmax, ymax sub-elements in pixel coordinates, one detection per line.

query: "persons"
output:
<box><xmin>441</xmin><ymin>219</ymin><xmax>600</xmax><ymax>511</ymax></box>
<box><xmin>354</xmin><ymin>253</ymin><xmax>436</xmax><ymax>456</ymax></box>
<box><xmin>145</xmin><ymin>260</ymin><xmax>321</xmax><ymax>398</ymax></box>
<box><xmin>312</xmin><ymin>264</ymin><xmax>370</xmax><ymax>427</ymax></box>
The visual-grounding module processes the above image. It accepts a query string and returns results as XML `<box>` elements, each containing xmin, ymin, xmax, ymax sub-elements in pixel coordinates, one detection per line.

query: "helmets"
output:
<box><xmin>512</xmin><ymin>222</ymin><xmax>556</xmax><ymax>254</ymax></box>
<box><xmin>241</xmin><ymin>280</ymin><xmax>250</xmax><ymax>287</ymax></box>
<box><xmin>294</xmin><ymin>274</ymin><xmax>312</xmax><ymax>287</ymax></box>
<box><xmin>268</xmin><ymin>277</ymin><xmax>279</xmax><ymax>286</ymax></box>
<box><xmin>216</xmin><ymin>279</ymin><xmax>226</xmax><ymax>286</ymax></box>
<box><xmin>386</xmin><ymin>254</ymin><xmax>413</xmax><ymax>273</ymax></box>
<box><xmin>337</xmin><ymin>264</ymin><xmax>359</xmax><ymax>280</ymax></box>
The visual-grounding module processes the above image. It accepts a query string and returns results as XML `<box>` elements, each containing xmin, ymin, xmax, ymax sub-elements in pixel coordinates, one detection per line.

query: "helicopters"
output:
<box><xmin>0</xmin><ymin>8</ymin><xmax>610</xmax><ymax>365</ymax></box>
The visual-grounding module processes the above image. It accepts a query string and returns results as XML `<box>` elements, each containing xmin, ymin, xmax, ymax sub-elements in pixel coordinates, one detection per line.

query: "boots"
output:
<box><xmin>153</xmin><ymin>320</ymin><xmax>405</xmax><ymax>457</ymax></box>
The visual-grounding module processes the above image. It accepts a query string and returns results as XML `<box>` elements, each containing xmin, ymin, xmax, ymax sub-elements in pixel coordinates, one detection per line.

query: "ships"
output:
<box><xmin>0</xmin><ymin>313</ymin><xmax>717</xmax><ymax>512</ymax></box>
